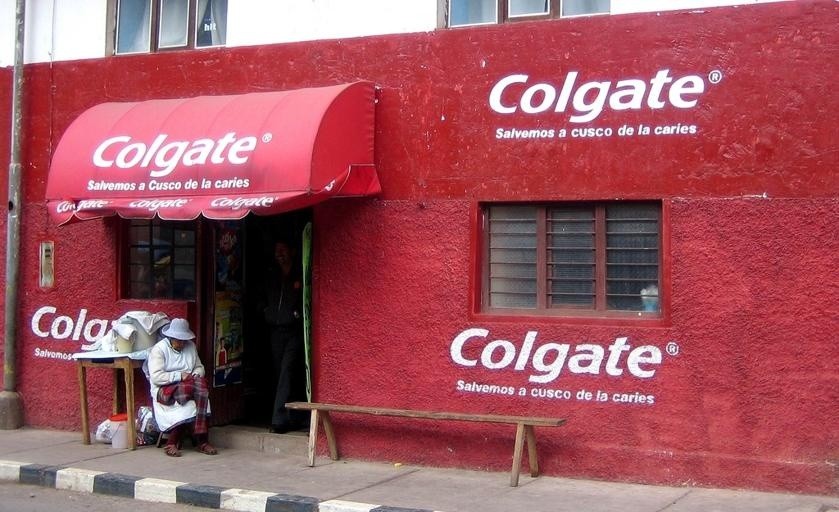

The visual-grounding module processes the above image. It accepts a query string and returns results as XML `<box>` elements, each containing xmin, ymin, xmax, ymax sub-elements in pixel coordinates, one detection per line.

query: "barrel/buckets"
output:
<box><xmin>108</xmin><ymin>414</ymin><xmax>129</xmax><ymax>449</ymax></box>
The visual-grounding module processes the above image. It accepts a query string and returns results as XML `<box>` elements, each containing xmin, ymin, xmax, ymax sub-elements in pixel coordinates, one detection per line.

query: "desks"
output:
<box><xmin>76</xmin><ymin>351</ymin><xmax>145</xmax><ymax>452</ymax></box>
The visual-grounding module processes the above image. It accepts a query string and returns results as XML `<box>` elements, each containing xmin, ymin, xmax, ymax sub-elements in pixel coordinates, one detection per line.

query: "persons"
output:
<box><xmin>146</xmin><ymin>317</ymin><xmax>218</xmax><ymax>457</ymax></box>
<box><xmin>252</xmin><ymin>239</ymin><xmax>303</xmax><ymax>433</ymax></box>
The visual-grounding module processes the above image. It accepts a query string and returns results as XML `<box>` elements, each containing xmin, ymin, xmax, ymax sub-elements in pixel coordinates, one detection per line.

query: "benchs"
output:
<box><xmin>284</xmin><ymin>401</ymin><xmax>568</xmax><ymax>487</ymax></box>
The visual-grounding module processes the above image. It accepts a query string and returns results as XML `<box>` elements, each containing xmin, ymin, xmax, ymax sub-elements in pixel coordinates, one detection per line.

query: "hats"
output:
<box><xmin>159</xmin><ymin>318</ymin><xmax>196</xmax><ymax>342</ymax></box>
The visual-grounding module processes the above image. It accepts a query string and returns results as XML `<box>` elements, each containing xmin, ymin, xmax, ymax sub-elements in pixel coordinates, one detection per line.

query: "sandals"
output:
<box><xmin>163</xmin><ymin>444</ymin><xmax>182</xmax><ymax>459</ymax></box>
<box><xmin>195</xmin><ymin>443</ymin><xmax>218</xmax><ymax>456</ymax></box>
